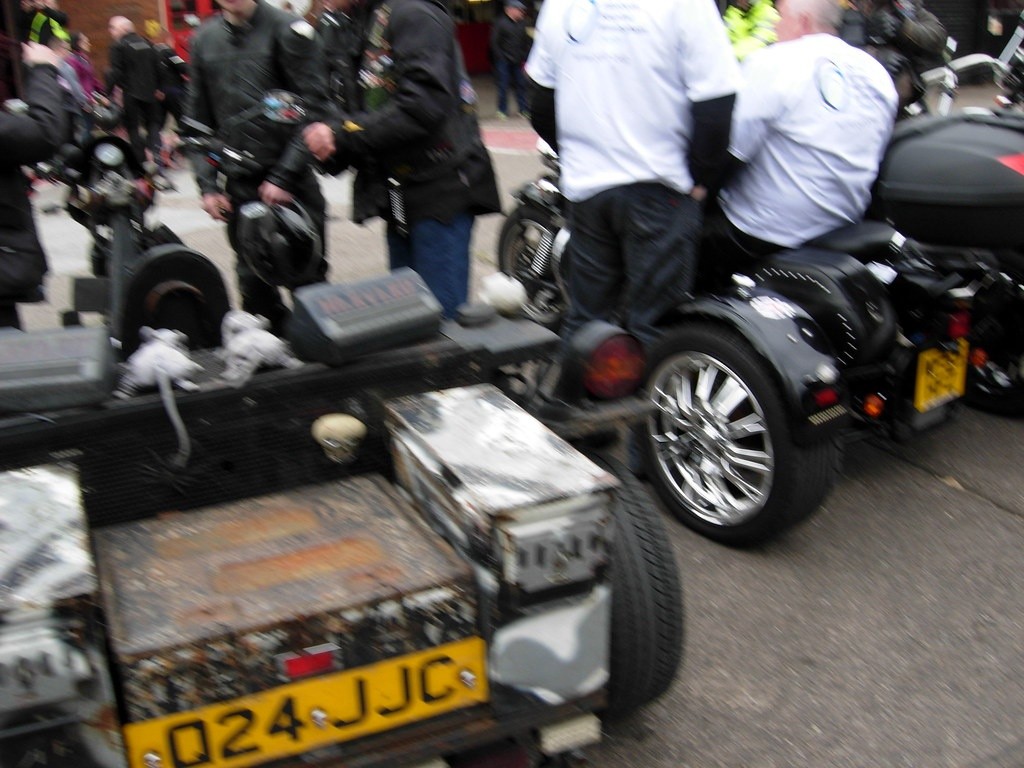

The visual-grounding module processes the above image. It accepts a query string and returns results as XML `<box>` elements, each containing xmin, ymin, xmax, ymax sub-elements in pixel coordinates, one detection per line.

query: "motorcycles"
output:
<box><xmin>495</xmin><ymin>21</ymin><xmax>1024</xmax><ymax>552</ymax></box>
<box><xmin>1</xmin><ymin>92</ymin><xmax>685</xmax><ymax>767</ymax></box>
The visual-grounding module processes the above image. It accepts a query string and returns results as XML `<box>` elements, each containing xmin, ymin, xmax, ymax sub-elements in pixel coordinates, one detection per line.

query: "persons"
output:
<box><xmin>307</xmin><ymin>1</ymin><xmax>504</xmax><ymax>313</ymax></box>
<box><xmin>515</xmin><ymin>0</ymin><xmax>737</xmax><ymax>344</ymax></box>
<box><xmin>183</xmin><ymin>1</ymin><xmax>336</xmax><ymax>317</ymax></box>
<box><xmin>490</xmin><ymin>0</ymin><xmax>527</xmax><ymax>116</ymax></box>
<box><xmin>695</xmin><ymin>1</ymin><xmax>897</xmax><ymax>296</ymax></box>
<box><xmin>0</xmin><ymin>36</ymin><xmax>85</xmax><ymax>329</ymax></box>
<box><xmin>66</xmin><ymin>18</ymin><xmax>187</xmax><ymax>188</ymax></box>
